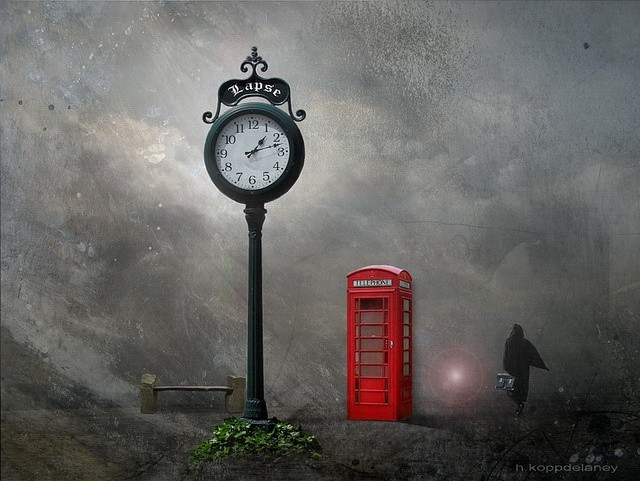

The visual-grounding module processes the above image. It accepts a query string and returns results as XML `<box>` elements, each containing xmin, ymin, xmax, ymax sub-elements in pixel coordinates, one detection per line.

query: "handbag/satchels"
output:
<box><xmin>494</xmin><ymin>373</ymin><xmax>520</xmax><ymax>394</ymax></box>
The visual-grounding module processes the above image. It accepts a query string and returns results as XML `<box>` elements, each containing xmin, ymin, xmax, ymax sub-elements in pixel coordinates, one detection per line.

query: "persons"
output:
<box><xmin>503</xmin><ymin>324</ymin><xmax>546</xmax><ymax>417</ymax></box>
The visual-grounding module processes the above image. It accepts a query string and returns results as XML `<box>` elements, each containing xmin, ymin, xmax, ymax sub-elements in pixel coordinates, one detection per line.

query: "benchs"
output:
<box><xmin>140</xmin><ymin>372</ymin><xmax>246</xmax><ymax>414</ymax></box>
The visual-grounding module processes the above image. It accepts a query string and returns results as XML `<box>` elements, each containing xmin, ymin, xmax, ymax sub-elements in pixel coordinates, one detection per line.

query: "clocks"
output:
<box><xmin>202</xmin><ymin>100</ymin><xmax>306</xmax><ymax>206</ymax></box>
